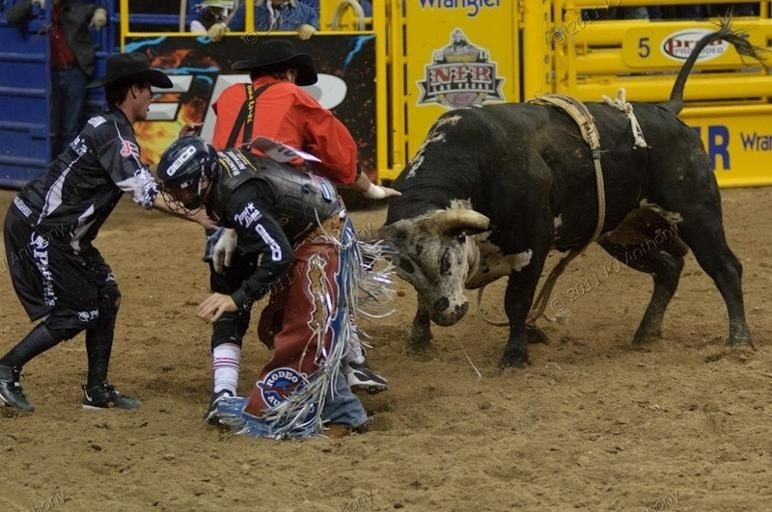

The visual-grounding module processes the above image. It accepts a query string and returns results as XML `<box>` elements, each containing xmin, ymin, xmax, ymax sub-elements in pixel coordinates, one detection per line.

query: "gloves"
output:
<box><xmin>208</xmin><ymin>23</ymin><xmax>229</xmax><ymax>42</ymax></box>
<box><xmin>212</xmin><ymin>228</ymin><xmax>238</xmax><ymax>274</ymax></box>
<box><xmin>296</xmin><ymin>24</ymin><xmax>316</xmax><ymax>40</ymax></box>
<box><xmin>362</xmin><ymin>183</ymin><xmax>402</xmax><ymax>199</ymax></box>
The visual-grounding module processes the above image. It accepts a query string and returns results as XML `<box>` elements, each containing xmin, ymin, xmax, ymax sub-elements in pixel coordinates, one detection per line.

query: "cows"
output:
<box><xmin>356</xmin><ymin>8</ymin><xmax>772</xmax><ymax>371</ymax></box>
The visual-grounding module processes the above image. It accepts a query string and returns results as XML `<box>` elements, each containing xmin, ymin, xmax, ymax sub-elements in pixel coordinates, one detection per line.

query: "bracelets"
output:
<box><xmin>363</xmin><ymin>183</ymin><xmax>386</xmax><ymax>199</ymax></box>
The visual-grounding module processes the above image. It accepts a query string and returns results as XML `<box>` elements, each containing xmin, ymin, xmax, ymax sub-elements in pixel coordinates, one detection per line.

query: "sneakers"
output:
<box><xmin>204</xmin><ymin>389</ymin><xmax>234</xmax><ymax>423</ymax></box>
<box><xmin>0</xmin><ymin>363</ymin><xmax>35</xmax><ymax>412</ymax></box>
<box><xmin>346</xmin><ymin>366</ymin><xmax>389</xmax><ymax>391</ymax></box>
<box><xmin>82</xmin><ymin>379</ymin><xmax>142</xmax><ymax>410</ymax></box>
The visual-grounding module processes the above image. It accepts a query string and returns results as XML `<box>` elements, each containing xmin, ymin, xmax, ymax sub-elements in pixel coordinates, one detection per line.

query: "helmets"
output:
<box><xmin>155</xmin><ymin>135</ymin><xmax>218</xmax><ymax>217</ymax></box>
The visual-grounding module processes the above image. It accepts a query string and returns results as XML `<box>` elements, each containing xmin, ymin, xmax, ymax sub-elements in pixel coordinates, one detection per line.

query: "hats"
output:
<box><xmin>230</xmin><ymin>38</ymin><xmax>318</xmax><ymax>86</ymax></box>
<box><xmin>86</xmin><ymin>52</ymin><xmax>173</xmax><ymax>88</ymax></box>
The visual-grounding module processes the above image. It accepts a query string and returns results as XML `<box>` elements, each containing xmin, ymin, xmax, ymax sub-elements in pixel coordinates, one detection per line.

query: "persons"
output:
<box><xmin>8</xmin><ymin>0</ymin><xmax>109</xmax><ymax>148</ymax></box>
<box><xmin>204</xmin><ymin>41</ymin><xmax>403</xmax><ymax>428</ymax></box>
<box><xmin>2</xmin><ymin>54</ymin><xmax>219</xmax><ymax>414</ymax></box>
<box><xmin>186</xmin><ymin>1</ymin><xmax>240</xmax><ymax>41</ymax></box>
<box><xmin>155</xmin><ymin>134</ymin><xmax>371</xmax><ymax>442</ymax></box>
<box><xmin>208</xmin><ymin>0</ymin><xmax>322</xmax><ymax>45</ymax></box>
